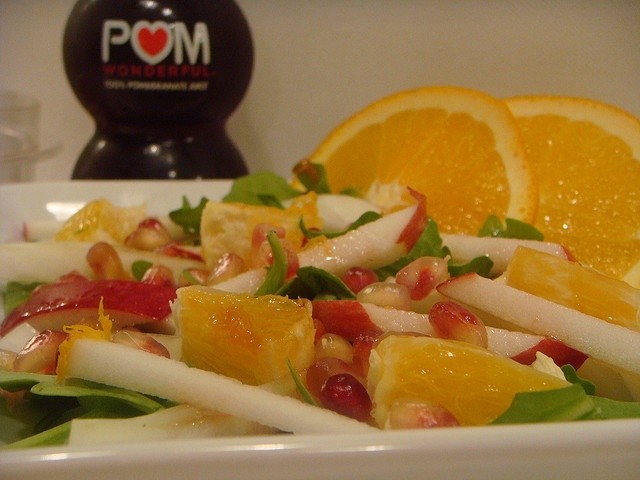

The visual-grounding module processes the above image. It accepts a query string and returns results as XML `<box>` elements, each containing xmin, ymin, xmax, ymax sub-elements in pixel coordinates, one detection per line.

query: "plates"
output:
<box><xmin>0</xmin><ymin>179</ymin><xmax>639</xmax><ymax>480</ymax></box>
